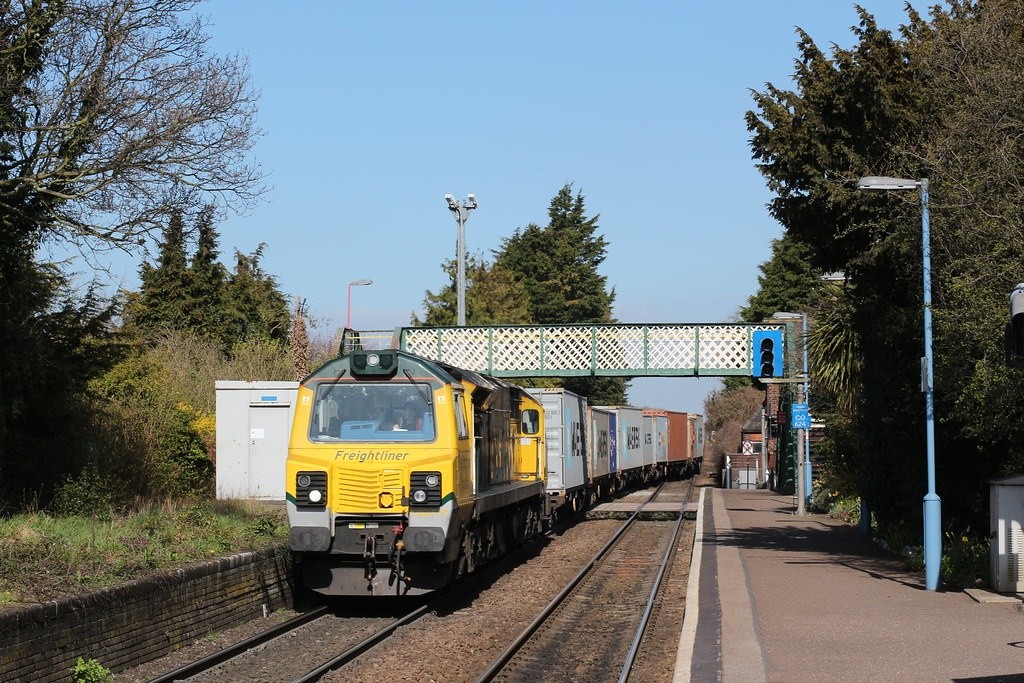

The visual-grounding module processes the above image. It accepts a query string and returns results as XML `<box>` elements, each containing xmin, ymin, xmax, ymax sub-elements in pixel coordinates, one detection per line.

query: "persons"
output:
<box><xmin>393</xmin><ymin>402</ymin><xmax>422</xmax><ymax>431</ymax></box>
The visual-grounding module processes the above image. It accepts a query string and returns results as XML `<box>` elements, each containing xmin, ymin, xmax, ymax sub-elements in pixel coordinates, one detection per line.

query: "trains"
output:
<box><xmin>284</xmin><ymin>348</ymin><xmax>706</xmax><ymax>600</ymax></box>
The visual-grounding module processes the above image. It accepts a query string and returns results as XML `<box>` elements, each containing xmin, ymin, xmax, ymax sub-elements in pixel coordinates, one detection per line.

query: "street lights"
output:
<box><xmin>443</xmin><ymin>193</ymin><xmax>479</xmax><ymax>325</ymax></box>
<box><xmin>772</xmin><ymin>311</ymin><xmax>813</xmax><ymax>511</ymax></box>
<box><xmin>345</xmin><ymin>278</ymin><xmax>373</xmax><ymax>328</ymax></box>
<box><xmin>856</xmin><ymin>175</ymin><xmax>944</xmax><ymax>591</ymax></box>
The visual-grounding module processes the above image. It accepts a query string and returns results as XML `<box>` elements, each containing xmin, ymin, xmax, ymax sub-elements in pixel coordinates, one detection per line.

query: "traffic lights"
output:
<box><xmin>751</xmin><ymin>329</ymin><xmax>784</xmax><ymax>378</ymax></box>
